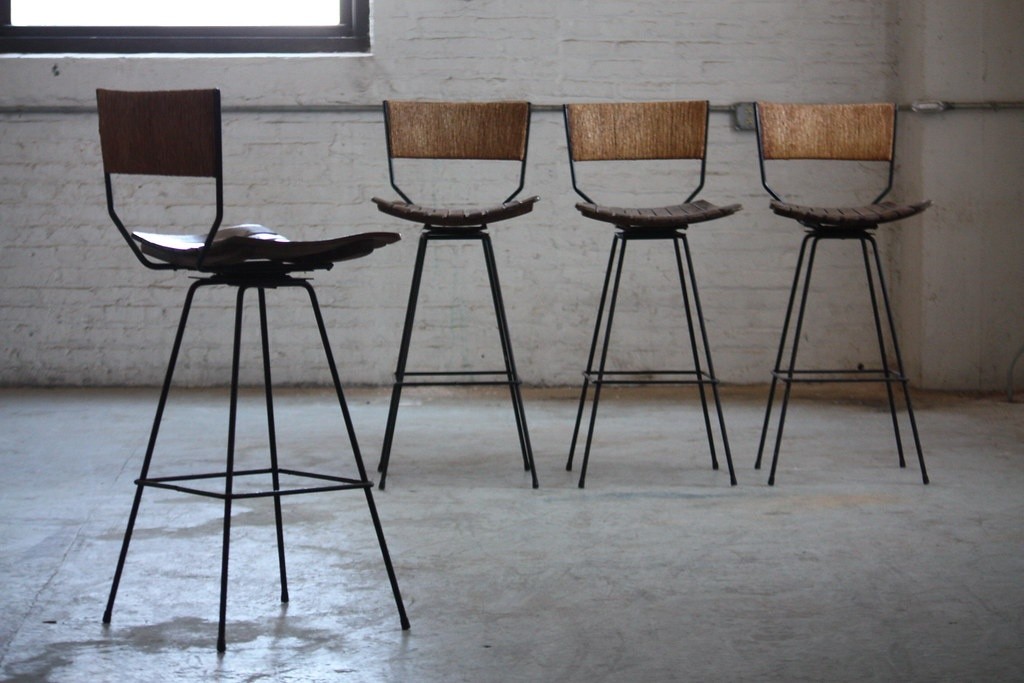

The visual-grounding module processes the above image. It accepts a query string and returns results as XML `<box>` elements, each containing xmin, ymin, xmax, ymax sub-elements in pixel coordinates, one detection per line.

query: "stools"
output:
<box><xmin>562</xmin><ymin>102</ymin><xmax>737</xmax><ymax>488</ymax></box>
<box><xmin>370</xmin><ymin>101</ymin><xmax>545</xmax><ymax>489</ymax></box>
<box><xmin>94</xmin><ymin>86</ymin><xmax>412</xmax><ymax>658</ymax></box>
<box><xmin>753</xmin><ymin>102</ymin><xmax>934</xmax><ymax>484</ymax></box>
